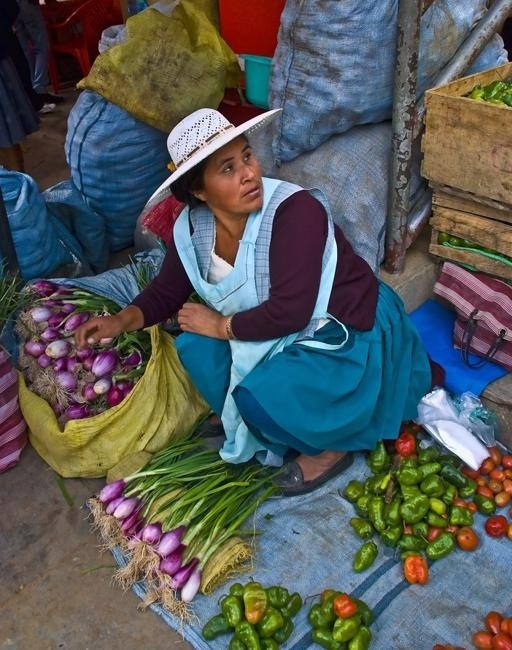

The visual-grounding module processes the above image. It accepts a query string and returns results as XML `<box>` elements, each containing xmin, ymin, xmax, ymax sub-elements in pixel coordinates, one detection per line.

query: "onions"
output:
<box><xmin>16</xmin><ymin>253</ymin><xmax>150</xmax><ymax>418</ymax></box>
<box><xmin>84</xmin><ymin>430</ymin><xmax>286</xmax><ymax>626</ymax></box>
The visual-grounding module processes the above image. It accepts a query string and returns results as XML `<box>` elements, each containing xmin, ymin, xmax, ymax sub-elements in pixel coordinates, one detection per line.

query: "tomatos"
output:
<box><xmin>430</xmin><ymin>612</ymin><xmax>512</xmax><ymax>650</ymax></box>
<box><xmin>456</xmin><ymin>445</ymin><xmax>512</xmax><ymax>550</ymax></box>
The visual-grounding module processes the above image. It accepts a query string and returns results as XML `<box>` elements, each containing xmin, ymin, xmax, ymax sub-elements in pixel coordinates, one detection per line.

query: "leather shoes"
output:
<box><xmin>194</xmin><ymin>412</ymin><xmax>225</xmax><ymax>438</ymax></box>
<box><xmin>263</xmin><ymin>450</ymin><xmax>355</xmax><ymax>496</ymax></box>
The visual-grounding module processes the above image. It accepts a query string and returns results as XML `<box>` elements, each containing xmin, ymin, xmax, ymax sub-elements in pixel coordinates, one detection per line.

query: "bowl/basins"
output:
<box><xmin>237</xmin><ymin>53</ymin><xmax>274</xmax><ymax>110</ymax></box>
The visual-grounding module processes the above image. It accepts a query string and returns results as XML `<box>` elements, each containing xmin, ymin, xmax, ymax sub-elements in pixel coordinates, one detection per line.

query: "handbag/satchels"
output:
<box><xmin>433</xmin><ymin>261</ymin><xmax>512</xmax><ymax>367</ymax></box>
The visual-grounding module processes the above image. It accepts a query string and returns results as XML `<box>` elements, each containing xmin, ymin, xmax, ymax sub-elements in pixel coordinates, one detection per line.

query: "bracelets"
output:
<box><xmin>225</xmin><ymin>315</ymin><xmax>235</xmax><ymax>339</ymax></box>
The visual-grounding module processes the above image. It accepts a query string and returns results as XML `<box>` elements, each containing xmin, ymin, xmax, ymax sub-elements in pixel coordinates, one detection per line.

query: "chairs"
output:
<box><xmin>40</xmin><ymin>0</ymin><xmax>123</xmax><ymax>91</ymax></box>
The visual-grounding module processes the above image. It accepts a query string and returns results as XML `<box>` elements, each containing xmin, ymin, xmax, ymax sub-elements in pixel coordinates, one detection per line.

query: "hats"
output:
<box><xmin>147</xmin><ymin>108</ymin><xmax>284</xmax><ymax>208</ymax></box>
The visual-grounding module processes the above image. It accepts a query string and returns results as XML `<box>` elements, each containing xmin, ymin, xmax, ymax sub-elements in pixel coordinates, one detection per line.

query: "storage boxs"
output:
<box><xmin>428</xmin><ymin>180</ymin><xmax>512</xmax><ymax>281</ymax></box>
<box><xmin>420</xmin><ymin>63</ymin><xmax>512</xmax><ymax>207</ymax></box>
<box><xmin>217</xmin><ymin>0</ymin><xmax>286</xmax><ymax>53</ymax></box>
<box><xmin>241</xmin><ymin>54</ymin><xmax>279</xmax><ymax>109</ymax></box>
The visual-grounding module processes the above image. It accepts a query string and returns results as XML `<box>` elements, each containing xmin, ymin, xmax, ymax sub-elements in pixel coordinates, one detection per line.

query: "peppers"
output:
<box><xmin>338</xmin><ymin>420</ymin><xmax>496</xmax><ymax>585</ymax></box>
<box><xmin>308</xmin><ymin>590</ymin><xmax>373</xmax><ymax>650</ymax></box>
<box><xmin>202</xmin><ymin>577</ymin><xmax>303</xmax><ymax>650</ymax></box>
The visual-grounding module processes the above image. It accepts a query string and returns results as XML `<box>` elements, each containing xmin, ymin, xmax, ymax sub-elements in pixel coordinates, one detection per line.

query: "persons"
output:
<box><xmin>0</xmin><ymin>0</ymin><xmax>40</xmax><ymax>174</ymax></box>
<box><xmin>12</xmin><ymin>0</ymin><xmax>64</xmax><ymax>113</ymax></box>
<box><xmin>76</xmin><ymin>108</ymin><xmax>433</xmax><ymax>496</ymax></box>
